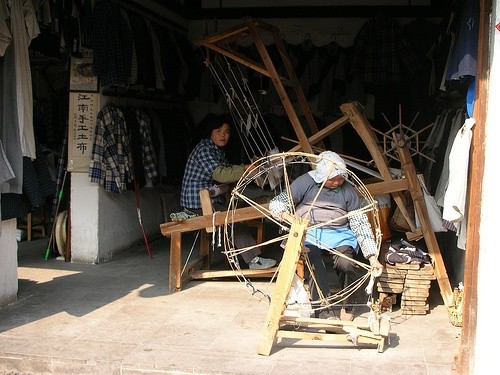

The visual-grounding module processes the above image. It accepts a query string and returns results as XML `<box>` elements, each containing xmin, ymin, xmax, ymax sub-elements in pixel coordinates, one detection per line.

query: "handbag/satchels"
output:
<box><xmin>390</xmin><ymin>191</ymin><xmax>415</xmax><ymax>233</ymax></box>
<box><xmin>414</xmin><ymin>175</ymin><xmax>448</xmax><ymax>232</ymax></box>
<box><xmin>360</xmin><ymin>194</ymin><xmax>392</xmax><ymax>241</ymax></box>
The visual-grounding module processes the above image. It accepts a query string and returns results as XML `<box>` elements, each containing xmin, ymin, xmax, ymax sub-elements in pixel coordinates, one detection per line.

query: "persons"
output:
<box><xmin>180</xmin><ymin>117</ymin><xmax>277</xmax><ymax>269</ymax></box>
<box><xmin>269</xmin><ymin>151</ymin><xmax>382</xmax><ymax>321</ymax></box>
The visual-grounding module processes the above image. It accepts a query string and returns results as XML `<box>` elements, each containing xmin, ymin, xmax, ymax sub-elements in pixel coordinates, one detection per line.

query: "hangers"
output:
<box><xmin>280</xmin><ymin>33</ymin><xmax>350</xmax><ymax>48</ymax></box>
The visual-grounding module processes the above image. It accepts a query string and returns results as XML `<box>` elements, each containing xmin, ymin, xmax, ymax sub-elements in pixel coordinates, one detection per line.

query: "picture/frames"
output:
<box><xmin>68</xmin><ymin>55</ymin><xmax>100</xmax><ymax>93</ymax></box>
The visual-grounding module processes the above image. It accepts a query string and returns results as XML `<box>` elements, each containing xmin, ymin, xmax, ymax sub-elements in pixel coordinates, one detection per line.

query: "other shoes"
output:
<box><xmin>249</xmin><ymin>257</ymin><xmax>277</xmax><ymax>270</ymax></box>
<box><xmin>319</xmin><ymin>307</ymin><xmax>334</xmax><ymax>318</ymax></box>
<box><xmin>339</xmin><ymin>305</ymin><xmax>355</xmax><ymax>321</ymax></box>
